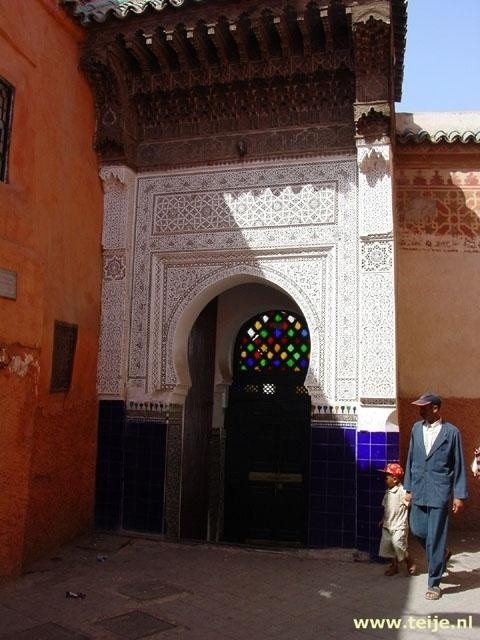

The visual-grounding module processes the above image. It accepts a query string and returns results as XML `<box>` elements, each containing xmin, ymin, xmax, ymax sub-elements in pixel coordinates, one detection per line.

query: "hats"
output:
<box><xmin>411</xmin><ymin>392</ymin><xmax>441</xmax><ymax>408</ymax></box>
<box><xmin>376</xmin><ymin>462</ymin><xmax>404</xmax><ymax>481</ymax></box>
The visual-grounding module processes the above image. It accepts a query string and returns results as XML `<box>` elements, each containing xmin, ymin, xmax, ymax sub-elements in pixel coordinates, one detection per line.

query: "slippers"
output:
<box><xmin>441</xmin><ymin>545</ymin><xmax>451</xmax><ymax>574</ymax></box>
<box><xmin>425</xmin><ymin>584</ymin><xmax>443</xmax><ymax>600</ymax></box>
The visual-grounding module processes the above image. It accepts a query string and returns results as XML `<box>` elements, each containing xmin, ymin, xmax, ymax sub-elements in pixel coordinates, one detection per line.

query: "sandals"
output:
<box><xmin>385</xmin><ymin>564</ymin><xmax>400</xmax><ymax>576</ymax></box>
<box><xmin>406</xmin><ymin>550</ymin><xmax>417</xmax><ymax>575</ymax></box>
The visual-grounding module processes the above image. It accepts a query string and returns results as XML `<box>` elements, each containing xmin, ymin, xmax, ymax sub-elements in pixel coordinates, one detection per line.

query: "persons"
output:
<box><xmin>402</xmin><ymin>392</ymin><xmax>470</xmax><ymax>601</ymax></box>
<box><xmin>376</xmin><ymin>461</ymin><xmax>418</xmax><ymax>577</ymax></box>
